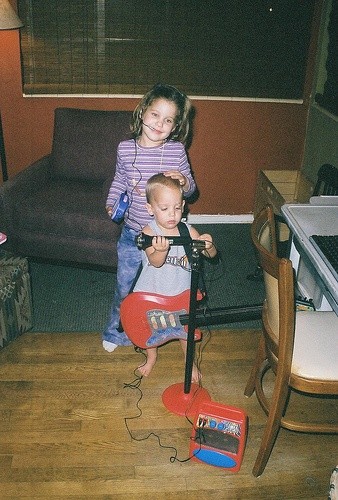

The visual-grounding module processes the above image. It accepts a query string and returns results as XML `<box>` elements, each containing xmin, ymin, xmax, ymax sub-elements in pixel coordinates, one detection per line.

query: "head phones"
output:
<box><xmin>138</xmin><ymin>82</ymin><xmax>186</xmax><ymax>135</ymax></box>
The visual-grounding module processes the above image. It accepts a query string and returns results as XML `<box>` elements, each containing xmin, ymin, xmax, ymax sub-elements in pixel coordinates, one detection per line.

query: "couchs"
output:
<box><xmin>0</xmin><ymin>108</ymin><xmax>140</xmax><ymax>269</ymax></box>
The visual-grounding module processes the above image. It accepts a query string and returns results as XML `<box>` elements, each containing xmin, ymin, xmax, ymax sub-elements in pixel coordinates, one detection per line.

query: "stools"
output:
<box><xmin>0</xmin><ymin>248</ymin><xmax>34</xmax><ymax>351</ymax></box>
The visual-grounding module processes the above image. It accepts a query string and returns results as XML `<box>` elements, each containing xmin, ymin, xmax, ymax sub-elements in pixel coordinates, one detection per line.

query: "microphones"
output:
<box><xmin>138</xmin><ymin>108</ymin><xmax>155</xmax><ymax>131</ymax></box>
<box><xmin>134</xmin><ymin>232</ymin><xmax>192</xmax><ymax>251</ymax></box>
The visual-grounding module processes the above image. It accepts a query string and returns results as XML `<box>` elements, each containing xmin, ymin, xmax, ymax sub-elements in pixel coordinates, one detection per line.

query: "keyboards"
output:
<box><xmin>309</xmin><ymin>235</ymin><xmax>338</xmax><ymax>281</ymax></box>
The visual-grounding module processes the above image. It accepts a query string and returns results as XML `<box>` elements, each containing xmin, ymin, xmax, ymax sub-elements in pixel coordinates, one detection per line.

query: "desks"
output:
<box><xmin>280</xmin><ymin>205</ymin><xmax>338</xmax><ymax>320</ymax></box>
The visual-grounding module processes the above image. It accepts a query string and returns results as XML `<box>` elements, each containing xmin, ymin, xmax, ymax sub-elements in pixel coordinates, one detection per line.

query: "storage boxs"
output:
<box><xmin>256</xmin><ymin>169</ymin><xmax>317</xmax><ymax>218</ymax></box>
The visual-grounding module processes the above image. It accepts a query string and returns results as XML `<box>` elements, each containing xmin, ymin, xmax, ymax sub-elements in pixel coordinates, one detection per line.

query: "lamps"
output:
<box><xmin>0</xmin><ymin>0</ymin><xmax>24</xmax><ymax>29</ymax></box>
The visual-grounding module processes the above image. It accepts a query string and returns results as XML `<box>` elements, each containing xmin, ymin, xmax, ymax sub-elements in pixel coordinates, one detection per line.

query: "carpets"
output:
<box><xmin>23</xmin><ymin>222</ymin><xmax>264</xmax><ymax>333</ymax></box>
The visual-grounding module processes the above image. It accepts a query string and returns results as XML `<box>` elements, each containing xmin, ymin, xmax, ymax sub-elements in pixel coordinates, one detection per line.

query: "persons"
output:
<box><xmin>100</xmin><ymin>82</ymin><xmax>198</xmax><ymax>353</ymax></box>
<box><xmin>133</xmin><ymin>173</ymin><xmax>217</xmax><ymax>384</ymax></box>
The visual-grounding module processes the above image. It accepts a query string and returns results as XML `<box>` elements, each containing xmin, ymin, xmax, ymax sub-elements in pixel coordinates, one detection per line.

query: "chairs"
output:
<box><xmin>244</xmin><ymin>205</ymin><xmax>338</xmax><ymax>477</ymax></box>
